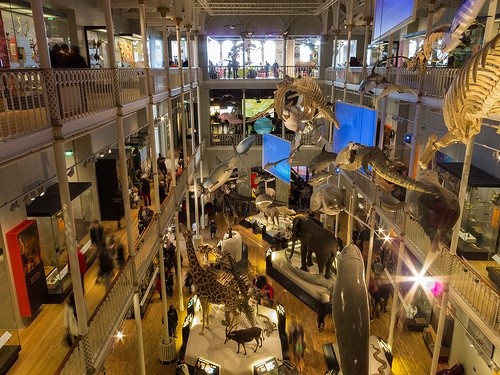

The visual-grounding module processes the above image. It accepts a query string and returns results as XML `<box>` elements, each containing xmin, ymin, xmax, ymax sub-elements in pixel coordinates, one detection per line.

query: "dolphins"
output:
<box><xmin>331</xmin><ymin>244</ymin><xmax>370</xmax><ymax>375</ymax></box>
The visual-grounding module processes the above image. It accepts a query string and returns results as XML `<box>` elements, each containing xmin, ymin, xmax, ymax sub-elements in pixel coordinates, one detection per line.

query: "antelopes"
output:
<box><xmin>224</xmin><ymin>321</ymin><xmax>263</xmax><ymax>356</ymax></box>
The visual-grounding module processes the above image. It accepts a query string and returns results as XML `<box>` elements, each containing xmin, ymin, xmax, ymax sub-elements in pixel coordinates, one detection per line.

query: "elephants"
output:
<box><xmin>289</xmin><ymin>215</ymin><xmax>340</xmax><ymax>279</ymax></box>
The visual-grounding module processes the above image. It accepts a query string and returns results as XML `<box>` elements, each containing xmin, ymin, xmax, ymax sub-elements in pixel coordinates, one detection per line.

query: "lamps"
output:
<box><xmin>30</xmin><ymin>192</ymin><xmax>35</xmax><ymax>201</ymax></box>
<box><xmin>37</xmin><ymin>187</ymin><xmax>45</xmax><ymax>197</ymax></box>
<box><xmin>83</xmin><ymin>160</ymin><xmax>88</xmax><ymax>168</ymax></box>
<box><xmin>126</xmin><ymin>135</ymin><xmax>131</xmax><ymax>143</ymax></box>
<box><xmin>10</xmin><ymin>200</ymin><xmax>20</xmax><ymax>213</ymax></box>
<box><xmin>254</xmin><ymin>97</ymin><xmax>261</xmax><ymax>104</ymax></box>
<box><xmin>108</xmin><ymin>146</ymin><xmax>112</xmax><ymax>154</ymax></box>
<box><xmin>92</xmin><ymin>155</ymin><xmax>98</xmax><ymax>162</ymax></box>
<box><xmin>67</xmin><ymin>166</ymin><xmax>75</xmax><ymax>178</ymax></box>
<box><xmin>492</xmin><ymin>150</ymin><xmax>500</xmax><ymax>161</ymax></box>
<box><xmin>88</xmin><ymin>158</ymin><xmax>92</xmax><ymax>164</ymax></box>
<box><xmin>99</xmin><ymin>149</ymin><xmax>105</xmax><ymax>158</ymax></box>
<box><xmin>32</xmin><ymin>190</ymin><xmax>37</xmax><ymax>198</ymax></box>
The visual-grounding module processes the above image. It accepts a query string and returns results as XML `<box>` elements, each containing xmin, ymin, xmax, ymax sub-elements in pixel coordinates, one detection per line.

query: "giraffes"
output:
<box><xmin>178</xmin><ymin>223</ymin><xmax>237</xmax><ymax>334</ymax></box>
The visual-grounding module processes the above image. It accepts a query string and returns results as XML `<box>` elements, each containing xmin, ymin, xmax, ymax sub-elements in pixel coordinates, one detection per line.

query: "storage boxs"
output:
<box><xmin>460</xmin><ymin>232</ymin><xmax>477</xmax><ymax>244</ymax></box>
<box><xmin>45</xmin><ymin>268</ymin><xmax>60</xmax><ymax>290</ymax></box>
<box><xmin>44</xmin><ymin>265</ymin><xmax>56</xmax><ymax>277</ymax></box>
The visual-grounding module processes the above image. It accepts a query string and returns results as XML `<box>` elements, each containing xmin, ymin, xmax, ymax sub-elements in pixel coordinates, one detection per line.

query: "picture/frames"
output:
<box><xmin>465</xmin><ymin>317</ymin><xmax>496</xmax><ymax>367</ymax></box>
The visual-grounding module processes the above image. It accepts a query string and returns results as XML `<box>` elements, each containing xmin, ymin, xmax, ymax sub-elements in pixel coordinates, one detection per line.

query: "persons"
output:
<box><xmin>209</xmin><ymin>219</ymin><xmax>217</xmax><ymax>239</ymax></box>
<box><xmin>352</xmin><ymin>227</ymin><xmax>368</xmax><ymax>246</ymax></box>
<box><xmin>228</xmin><ymin>58</ymin><xmax>239</xmax><ymax>78</ymax></box>
<box><xmin>90</xmin><ymin>220</ymin><xmax>125</xmax><ymax>288</ymax></box>
<box><xmin>385</xmin><ymin>161</ymin><xmax>407</xmax><ymax>200</ymax></box>
<box><xmin>157</xmin><ymin>244</ymin><xmax>183</xmax><ymax>300</ymax></box>
<box><xmin>178</xmin><ymin>155</ymin><xmax>189</xmax><ymax>167</ymax></box>
<box><xmin>157</xmin><ymin>154</ymin><xmax>167</xmax><ymax>175</ymax></box>
<box><xmin>370</xmin><ymin>277</ymin><xmax>391</xmax><ymax>321</ymax></box>
<box><xmin>66</xmin><ymin>246</ymin><xmax>85</xmax><ymax>297</ymax></box>
<box><xmin>138</xmin><ymin>204</ymin><xmax>154</xmax><ymax>224</ymax></box>
<box><xmin>273</xmin><ymin>60</ymin><xmax>278</xmax><ymax>77</ymax></box>
<box><xmin>141</xmin><ymin>177</ymin><xmax>151</xmax><ymax>206</ymax></box>
<box><xmin>192</xmin><ymin>221</ymin><xmax>200</xmax><ymax>235</ymax></box>
<box><xmin>252</xmin><ymin>275</ymin><xmax>266</xmax><ymax>304</ymax></box>
<box><xmin>290</xmin><ymin>180</ymin><xmax>310</xmax><ymax>208</ymax></box>
<box><xmin>262</xmin><ymin>284</ymin><xmax>274</xmax><ymax>308</ymax></box>
<box><xmin>168</xmin><ymin>305</ymin><xmax>178</xmax><ymax>337</ymax></box>
<box><xmin>159</xmin><ymin>181</ymin><xmax>165</xmax><ymax>204</ymax></box>
<box><xmin>289</xmin><ymin>324</ymin><xmax>305</xmax><ymax>365</ymax></box>
<box><xmin>336</xmin><ymin>236</ymin><xmax>343</xmax><ymax>250</ymax></box>
<box><xmin>64</xmin><ymin>292</ymin><xmax>87</xmax><ymax>344</ymax></box>
<box><xmin>265</xmin><ymin>61</ymin><xmax>270</xmax><ymax>76</ymax></box>
<box><xmin>50</xmin><ymin>43</ymin><xmax>89</xmax><ymax>117</ymax></box>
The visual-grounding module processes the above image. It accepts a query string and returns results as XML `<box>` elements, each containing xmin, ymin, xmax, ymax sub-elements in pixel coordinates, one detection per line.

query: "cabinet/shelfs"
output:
<box><xmin>0</xmin><ymin>247</ymin><xmax>22</xmax><ymax>375</ymax></box>
<box><xmin>96</xmin><ymin>142</ymin><xmax>135</xmax><ymax>221</ymax></box>
<box><xmin>25</xmin><ymin>180</ymin><xmax>101</xmax><ymax>305</ymax></box>
<box><xmin>401</xmin><ymin>26</ymin><xmax>456</xmax><ymax>68</ymax></box>
<box><xmin>0</xmin><ymin>0</ymin><xmax>71</xmax><ymax>111</ymax></box>
<box><xmin>84</xmin><ymin>25</ymin><xmax>118</xmax><ymax>94</ymax></box>
<box><xmin>404</xmin><ymin>296</ymin><xmax>432</xmax><ymax>332</ymax></box>
<box><xmin>114</xmin><ymin>32</ymin><xmax>150</xmax><ymax>89</ymax></box>
<box><xmin>435</xmin><ymin>161</ymin><xmax>500</xmax><ymax>262</ymax></box>
<box><xmin>421</xmin><ymin>299</ymin><xmax>456</xmax><ymax>365</ymax></box>
<box><xmin>366</xmin><ymin>40</ymin><xmax>400</xmax><ymax>86</ymax></box>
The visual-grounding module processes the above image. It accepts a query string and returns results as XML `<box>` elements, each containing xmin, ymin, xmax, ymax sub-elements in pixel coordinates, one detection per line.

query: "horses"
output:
<box><xmin>263</xmin><ymin>207</ymin><xmax>280</xmax><ymax>230</ymax></box>
